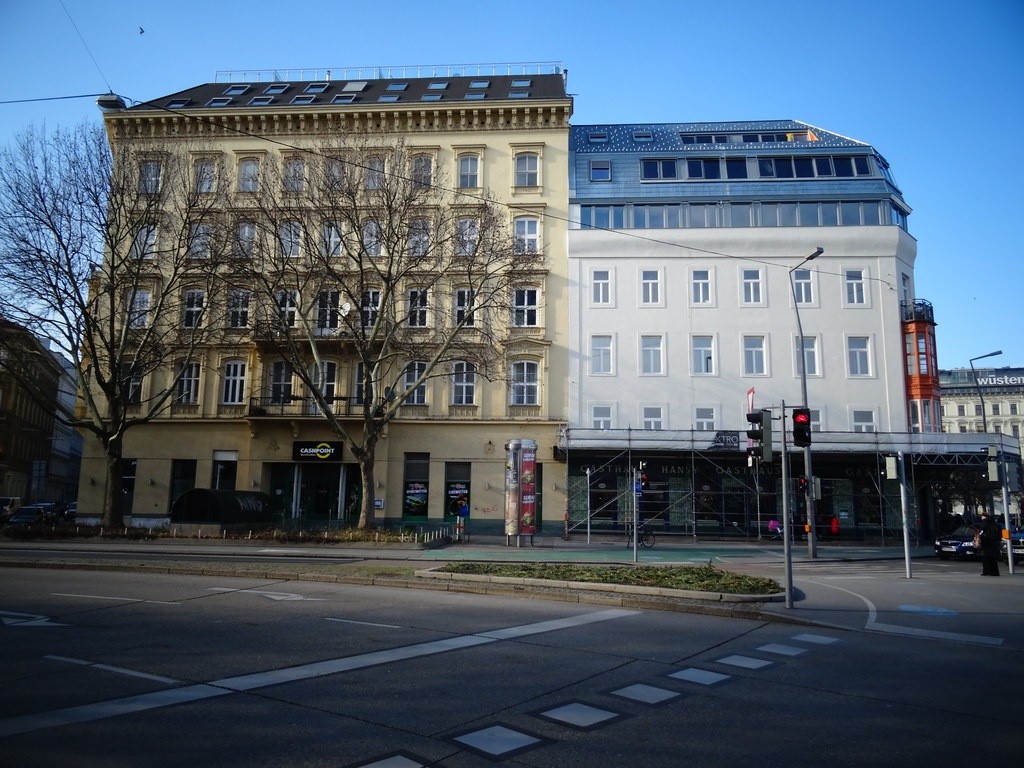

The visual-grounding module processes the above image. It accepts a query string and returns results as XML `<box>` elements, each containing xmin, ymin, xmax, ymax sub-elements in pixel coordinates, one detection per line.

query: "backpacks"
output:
<box><xmin>459</xmin><ymin>503</ymin><xmax>468</xmax><ymax>517</ymax></box>
<box><xmin>449</xmin><ymin>499</ymin><xmax>459</xmax><ymax>513</ymax></box>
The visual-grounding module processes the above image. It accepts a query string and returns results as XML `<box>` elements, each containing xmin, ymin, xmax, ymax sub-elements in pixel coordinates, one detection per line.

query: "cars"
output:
<box><xmin>0</xmin><ymin>496</ymin><xmax>22</xmax><ymax>524</ymax></box>
<box><xmin>995</xmin><ymin>522</ymin><xmax>1024</xmax><ymax>565</ymax></box>
<box><xmin>9</xmin><ymin>506</ymin><xmax>45</xmax><ymax>526</ymax></box>
<box><xmin>64</xmin><ymin>502</ymin><xmax>77</xmax><ymax>521</ymax></box>
<box><xmin>32</xmin><ymin>503</ymin><xmax>59</xmax><ymax>524</ymax></box>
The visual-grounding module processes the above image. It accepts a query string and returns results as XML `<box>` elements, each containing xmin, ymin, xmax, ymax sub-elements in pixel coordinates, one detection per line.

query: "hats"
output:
<box><xmin>979</xmin><ymin>512</ymin><xmax>990</xmax><ymax>518</ymax></box>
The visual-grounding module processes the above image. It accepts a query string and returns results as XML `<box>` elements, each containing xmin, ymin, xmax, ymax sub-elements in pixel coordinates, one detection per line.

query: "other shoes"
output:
<box><xmin>981</xmin><ymin>573</ymin><xmax>999</xmax><ymax>576</ymax></box>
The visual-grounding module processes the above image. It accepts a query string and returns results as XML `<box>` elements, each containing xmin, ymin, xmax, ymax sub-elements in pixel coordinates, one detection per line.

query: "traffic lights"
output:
<box><xmin>793</xmin><ymin>408</ymin><xmax>811</xmax><ymax>447</ymax></box>
<box><xmin>746</xmin><ymin>409</ymin><xmax>773</xmax><ymax>462</ymax></box>
<box><xmin>798</xmin><ymin>475</ymin><xmax>808</xmax><ymax>494</ymax></box>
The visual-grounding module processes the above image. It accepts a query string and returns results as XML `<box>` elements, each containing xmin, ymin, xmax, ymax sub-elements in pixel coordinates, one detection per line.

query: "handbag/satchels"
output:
<box><xmin>973</xmin><ymin>529</ymin><xmax>981</xmax><ymax>549</ymax></box>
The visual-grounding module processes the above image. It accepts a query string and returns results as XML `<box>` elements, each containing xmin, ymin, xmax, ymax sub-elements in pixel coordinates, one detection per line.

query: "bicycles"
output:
<box><xmin>624</xmin><ymin>522</ymin><xmax>655</xmax><ymax>548</ymax></box>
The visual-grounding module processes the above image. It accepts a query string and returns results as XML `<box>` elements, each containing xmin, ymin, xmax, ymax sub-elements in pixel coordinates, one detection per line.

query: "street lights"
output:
<box><xmin>789</xmin><ymin>247</ymin><xmax>826</xmax><ymax>559</ymax></box>
<box><xmin>934</xmin><ymin>525</ymin><xmax>983</xmax><ymax>562</ymax></box>
<box><xmin>968</xmin><ymin>348</ymin><xmax>1003</xmax><ymax>434</ymax></box>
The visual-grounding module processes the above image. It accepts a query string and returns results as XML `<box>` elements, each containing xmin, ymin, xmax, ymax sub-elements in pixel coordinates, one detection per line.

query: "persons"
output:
<box><xmin>831</xmin><ymin>514</ymin><xmax>839</xmax><ymax>533</ymax></box>
<box><xmin>976</xmin><ymin>512</ymin><xmax>1000</xmax><ymax>576</ymax></box>
